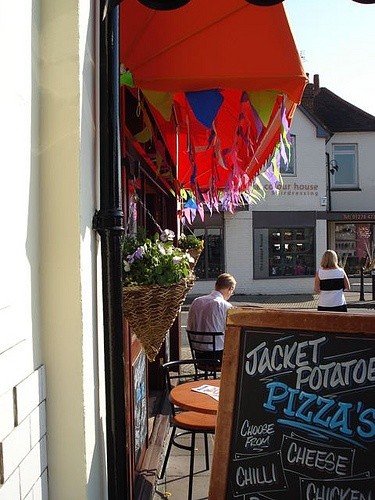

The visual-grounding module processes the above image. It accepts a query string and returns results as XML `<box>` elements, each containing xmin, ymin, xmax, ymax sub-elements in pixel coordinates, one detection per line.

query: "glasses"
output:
<box><xmin>232</xmin><ymin>289</ymin><xmax>234</xmax><ymax>295</ymax></box>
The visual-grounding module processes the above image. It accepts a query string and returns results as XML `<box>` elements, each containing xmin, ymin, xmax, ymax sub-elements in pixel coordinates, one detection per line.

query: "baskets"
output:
<box><xmin>122</xmin><ymin>273</ymin><xmax>195</xmax><ymax>362</ymax></box>
<box><xmin>181</xmin><ymin>248</ymin><xmax>202</xmax><ymax>272</ymax></box>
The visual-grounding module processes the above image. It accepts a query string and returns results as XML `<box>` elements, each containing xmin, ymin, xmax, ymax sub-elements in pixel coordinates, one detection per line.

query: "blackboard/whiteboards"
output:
<box><xmin>209</xmin><ymin>308</ymin><xmax>375</xmax><ymax>500</ymax></box>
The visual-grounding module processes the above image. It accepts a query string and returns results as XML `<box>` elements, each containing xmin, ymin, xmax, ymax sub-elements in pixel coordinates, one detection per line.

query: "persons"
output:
<box><xmin>314</xmin><ymin>250</ymin><xmax>350</xmax><ymax>313</ymax></box>
<box><xmin>187</xmin><ymin>273</ymin><xmax>237</xmax><ymax>366</ymax></box>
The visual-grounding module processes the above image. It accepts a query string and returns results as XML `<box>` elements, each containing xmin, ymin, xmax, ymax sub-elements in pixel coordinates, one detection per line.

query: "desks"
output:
<box><xmin>170</xmin><ymin>380</ymin><xmax>224</xmax><ymax>414</ymax></box>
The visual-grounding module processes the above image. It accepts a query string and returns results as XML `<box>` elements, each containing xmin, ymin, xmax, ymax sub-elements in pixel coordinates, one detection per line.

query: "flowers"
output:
<box><xmin>120</xmin><ymin>228</ymin><xmax>196</xmax><ymax>287</ymax></box>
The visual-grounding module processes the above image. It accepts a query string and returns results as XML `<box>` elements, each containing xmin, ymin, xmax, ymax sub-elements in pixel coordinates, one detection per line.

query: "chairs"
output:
<box><xmin>186</xmin><ymin>330</ymin><xmax>224</xmax><ymax>379</ymax></box>
<box><xmin>159</xmin><ymin>356</ymin><xmax>218</xmax><ymax>500</ymax></box>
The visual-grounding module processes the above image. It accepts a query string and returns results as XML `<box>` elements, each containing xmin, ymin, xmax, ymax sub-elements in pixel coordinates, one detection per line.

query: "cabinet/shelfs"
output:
<box><xmin>269</xmin><ymin>228</ymin><xmax>313</xmax><ymax>276</ymax></box>
